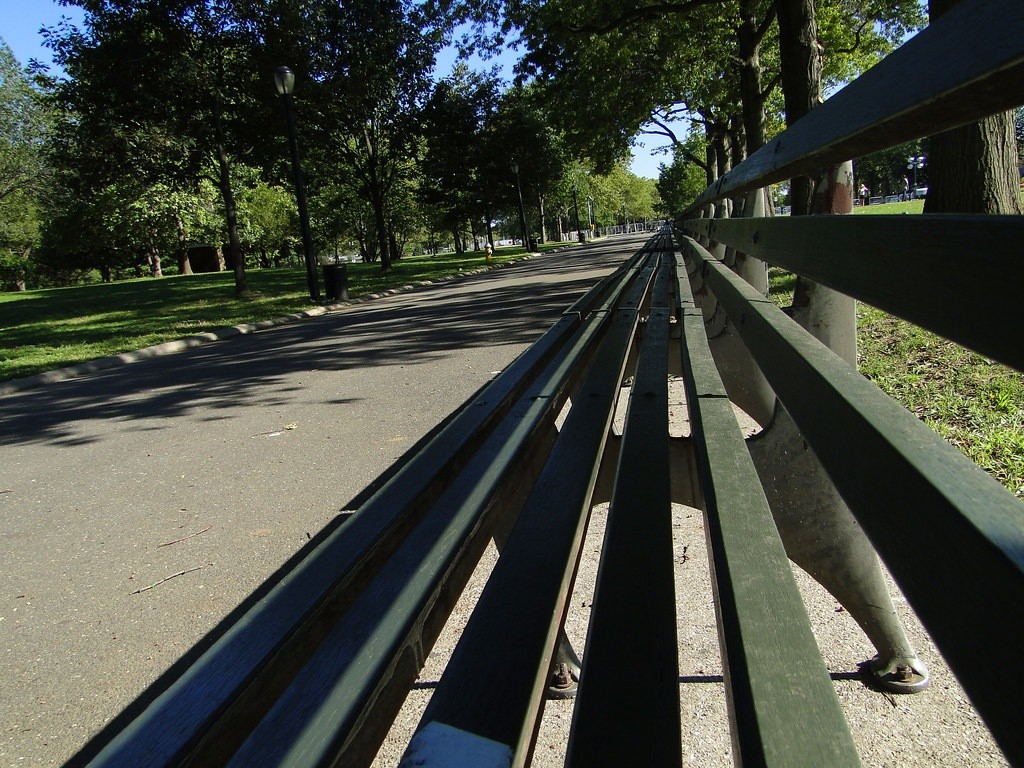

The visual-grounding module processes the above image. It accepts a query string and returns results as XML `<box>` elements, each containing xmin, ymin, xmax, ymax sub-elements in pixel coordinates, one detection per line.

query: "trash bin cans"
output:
<box><xmin>579</xmin><ymin>233</ymin><xmax>585</xmax><ymax>242</ymax></box>
<box><xmin>531</xmin><ymin>239</ymin><xmax>537</xmax><ymax>251</ymax></box>
<box><xmin>323</xmin><ymin>262</ymin><xmax>348</xmax><ymax>302</ymax></box>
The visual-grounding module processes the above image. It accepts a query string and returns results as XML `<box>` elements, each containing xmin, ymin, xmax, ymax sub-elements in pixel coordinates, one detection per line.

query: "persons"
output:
<box><xmin>901</xmin><ymin>174</ymin><xmax>911</xmax><ymax>201</ymax></box>
<box><xmin>858</xmin><ymin>182</ymin><xmax>868</xmax><ymax>206</ymax></box>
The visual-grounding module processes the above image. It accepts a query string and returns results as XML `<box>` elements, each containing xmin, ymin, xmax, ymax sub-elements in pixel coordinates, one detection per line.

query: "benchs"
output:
<box><xmin>82</xmin><ymin>0</ymin><xmax>1024</xmax><ymax>768</ymax></box>
